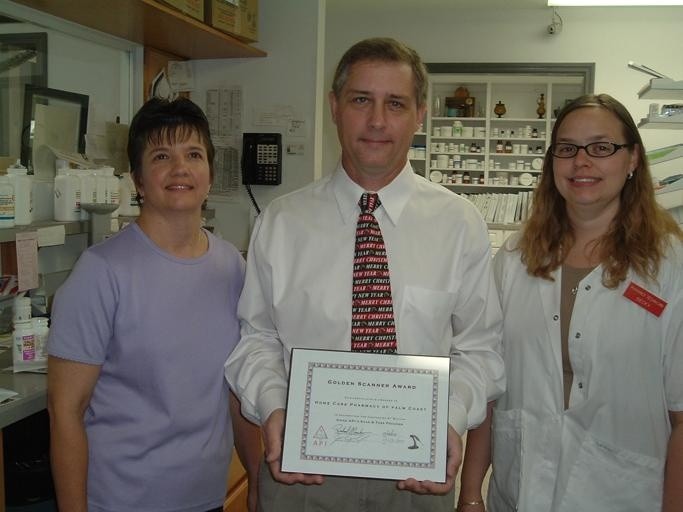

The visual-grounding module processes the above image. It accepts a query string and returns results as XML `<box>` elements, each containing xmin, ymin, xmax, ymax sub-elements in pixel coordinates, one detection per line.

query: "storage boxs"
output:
<box><xmin>156</xmin><ymin>0</ymin><xmax>260</xmax><ymax>45</ymax></box>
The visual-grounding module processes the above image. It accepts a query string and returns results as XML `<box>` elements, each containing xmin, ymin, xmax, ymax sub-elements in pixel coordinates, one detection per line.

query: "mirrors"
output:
<box><xmin>2</xmin><ymin>0</ymin><xmax>143</xmax><ymax>185</ymax></box>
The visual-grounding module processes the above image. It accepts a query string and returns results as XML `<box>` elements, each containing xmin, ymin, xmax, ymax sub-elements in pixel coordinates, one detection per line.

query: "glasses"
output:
<box><xmin>547</xmin><ymin>141</ymin><xmax>634</xmax><ymax>159</ymax></box>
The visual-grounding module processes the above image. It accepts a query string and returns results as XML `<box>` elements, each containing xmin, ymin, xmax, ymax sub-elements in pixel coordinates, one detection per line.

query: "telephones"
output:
<box><xmin>242</xmin><ymin>133</ymin><xmax>282</xmax><ymax>186</ymax></box>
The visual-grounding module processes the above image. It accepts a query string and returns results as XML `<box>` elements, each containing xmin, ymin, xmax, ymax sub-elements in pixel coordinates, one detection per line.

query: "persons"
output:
<box><xmin>455</xmin><ymin>93</ymin><xmax>682</xmax><ymax>511</ymax></box>
<box><xmin>224</xmin><ymin>36</ymin><xmax>508</xmax><ymax>512</ymax></box>
<box><xmin>41</xmin><ymin>94</ymin><xmax>263</xmax><ymax>512</ymax></box>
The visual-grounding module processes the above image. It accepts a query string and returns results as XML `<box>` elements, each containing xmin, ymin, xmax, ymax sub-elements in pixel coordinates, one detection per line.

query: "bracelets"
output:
<box><xmin>457</xmin><ymin>493</ymin><xmax>486</xmax><ymax>506</ymax></box>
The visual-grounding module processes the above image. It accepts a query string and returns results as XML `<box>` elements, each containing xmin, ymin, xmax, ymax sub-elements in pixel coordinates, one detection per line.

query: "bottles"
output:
<box><xmin>0</xmin><ymin>167</ymin><xmax>120</xmax><ymax>230</ymax></box>
<box><xmin>11</xmin><ymin>296</ymin><xmax>50</xmax><ymax>366</ymax></box>
<box><xmin>429</xmin><ymin>94</ymin><xmax>545</xmax><ymax>186</ymax></box>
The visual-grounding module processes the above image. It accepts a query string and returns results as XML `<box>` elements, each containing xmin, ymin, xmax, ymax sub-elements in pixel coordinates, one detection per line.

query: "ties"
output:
<box><xmin>350</xmin><ymin>193</ymin><xmax>398</xmax><ymax>354</ymax></box>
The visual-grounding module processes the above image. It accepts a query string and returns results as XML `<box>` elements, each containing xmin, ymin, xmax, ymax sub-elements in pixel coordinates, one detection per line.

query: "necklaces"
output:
<box><xmin>570</xmin><ymin>266</ymin><xmax>589</xmax><ymax>296</ymax></box>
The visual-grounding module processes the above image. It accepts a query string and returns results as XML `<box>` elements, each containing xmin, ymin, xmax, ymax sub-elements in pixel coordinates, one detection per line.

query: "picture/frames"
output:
<box><xmin>22</xmin><ymin>82</ymin><xmax>89</xmax><ymax>175</ymax></box>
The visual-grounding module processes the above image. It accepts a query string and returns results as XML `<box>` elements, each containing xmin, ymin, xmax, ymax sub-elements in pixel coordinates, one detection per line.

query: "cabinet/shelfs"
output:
<box><xmin>418</xmin><ymin>61</ymin><xmax>598</xmax><ymax>188</ymax></box>
<box><xmin>636</xmin><ymin>79</ymin><xmax>683</xmax><ymax>197</ymax></box>
